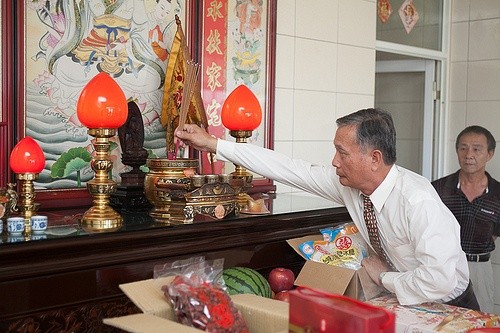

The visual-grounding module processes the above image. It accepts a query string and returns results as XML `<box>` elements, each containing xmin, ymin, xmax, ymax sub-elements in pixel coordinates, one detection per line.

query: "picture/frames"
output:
<box><xmin>14</xmin><ymin>0</ymin><xmax>277</xmax><ymax>201</ymax></box>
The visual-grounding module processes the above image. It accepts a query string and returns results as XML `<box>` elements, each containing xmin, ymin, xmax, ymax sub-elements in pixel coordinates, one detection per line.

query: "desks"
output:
<box><xmin>361</xmin><ymin>292</ymin><xmax>500</xmax><ymax>333</ymax></box>
<box><xmin>0</xmin><ymin>193</ymin><xmax>354</xmax><ymax>333</ymax></box>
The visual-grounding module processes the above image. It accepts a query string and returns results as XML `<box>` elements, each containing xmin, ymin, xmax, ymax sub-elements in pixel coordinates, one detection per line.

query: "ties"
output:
<box><xmin>361</xmin><ymin>193</ymin><xmax>398</xmax><ymax>272</ymax></box>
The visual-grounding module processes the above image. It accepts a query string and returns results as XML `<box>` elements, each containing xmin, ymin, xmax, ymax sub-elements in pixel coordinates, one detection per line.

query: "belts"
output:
<box><xmin>453</xmin><ymin>280</ymin><xmax>474</xmax><ymax>307</ymax></box>
<box><xmin>466</xmin><ymin>252</ymin><xmax>491</xmax><ymax>263</ymax></box>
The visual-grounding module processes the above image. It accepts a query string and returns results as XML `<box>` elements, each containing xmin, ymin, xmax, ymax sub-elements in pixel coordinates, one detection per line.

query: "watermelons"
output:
<box><xmin>222</xmin><ymin>266</ymin><xmax>272</xmax><ymax>299</ymax></box>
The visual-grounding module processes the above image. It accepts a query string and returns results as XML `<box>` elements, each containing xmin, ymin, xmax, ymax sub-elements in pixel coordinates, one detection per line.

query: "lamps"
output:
<box><xmin>221</xmin><ymin>84</ymin><xmax>262</xmax><ymax>209</ymax></box>
<box><xmin>9</xmin><ymin>136</ymin><xmax>45</xmax><ymax>232</ymax></box>
<box><xmin>77</xmin><ymin>72</ymin><xmax>129</xmax><ymax>229</ymax></box>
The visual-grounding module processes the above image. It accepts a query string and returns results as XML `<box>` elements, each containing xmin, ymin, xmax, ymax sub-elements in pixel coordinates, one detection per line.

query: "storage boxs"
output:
<box><xmin>285</xmin><ymin>234</ymin><xmax>384</xmax><ymax>300</ymax></box>
<box><xmin>289</xmin><ymin>289</ymin><xmax>396</xmax><ymax>333</ymax></box>
<box><xmin>103</xmin><ymin>274</ymin><xmax>290</xmax><ymax>333</ymax></box>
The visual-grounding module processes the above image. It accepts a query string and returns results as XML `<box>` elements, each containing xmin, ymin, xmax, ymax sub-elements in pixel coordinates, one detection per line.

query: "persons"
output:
<box><xmin>429</xmin><ymin>125</ymin><xmax>500</xmax><ymax>315</ymax></box>
<box><xmin>174</xmin><ymin>108</ymin><xmax>482</xmax><ymax>312</ymax></box>
<box><xmin>124</xmin><ymin>116</ymin><xmax>147</xmax><ymax>155</ymax></box>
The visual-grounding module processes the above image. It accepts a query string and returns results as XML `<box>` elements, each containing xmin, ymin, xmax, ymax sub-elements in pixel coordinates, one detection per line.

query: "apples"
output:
<box><xmin>268</xmin><ymin>267</ymin><xmax>295</xmax><ymax>301</ymax></box>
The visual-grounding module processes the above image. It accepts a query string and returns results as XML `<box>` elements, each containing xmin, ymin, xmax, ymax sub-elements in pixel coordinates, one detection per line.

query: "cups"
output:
<box><xmin>30</xmin><ymin>232</ymin><xmax>48</xmax><ymax>241</ymax></box>
<box><xmin>0</xmin><ymin>220</ymin><xmax>3</xmax><ymax>233</ymax></box>
<box><xmin>6</xmin><ymin>217</ymin><xmax>25</xmax><ymax>234</ymax></box>
<box><xmin>30</xmin><ymin>216</ymin><xmax>48</xmax><ymax>232</ymax></box>
<box><xmin>6</xmin><ymin>235</ymin><xmax>25</xmax><ymax>242</ymax></box>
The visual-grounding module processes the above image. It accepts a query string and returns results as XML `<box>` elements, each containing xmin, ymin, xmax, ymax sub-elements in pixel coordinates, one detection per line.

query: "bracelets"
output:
<box><xmin>379</xmin><ymin>270</ymin><xmax>390</xmax><ymax>294</ymax></box>
